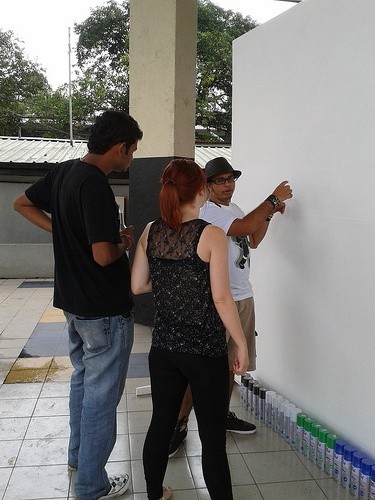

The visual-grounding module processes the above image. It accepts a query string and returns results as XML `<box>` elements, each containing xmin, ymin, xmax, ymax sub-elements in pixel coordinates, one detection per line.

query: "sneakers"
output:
<box><xmin>225</xmin><ymin>411</ymin><xmax>257</xmax><ymax>434</ymax></box>
<box><xmin>147</xmin><ymin>486</ymin><xmax>172</xmax><ymax>500</ymax></box>
<box><xmin>97</xmin><ymin>473</ymin><xmax>131</xmax><ymax>500</ymax></box>
<box><xmin>167</xmin><ymin>416</ymin><xmax>188</xmax><ymax>458</ymax></box>
<box><xmin>68</xmin><ymin>465</ymin><xmax>77</xmax><ymax>471</ymax></box>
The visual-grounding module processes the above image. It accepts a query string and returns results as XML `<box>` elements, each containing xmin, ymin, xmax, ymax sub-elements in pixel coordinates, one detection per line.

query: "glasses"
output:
<box><xmin>207</xmin><ymin>173</ymin><xmax>239</xmax><ymax>185</ymax></box>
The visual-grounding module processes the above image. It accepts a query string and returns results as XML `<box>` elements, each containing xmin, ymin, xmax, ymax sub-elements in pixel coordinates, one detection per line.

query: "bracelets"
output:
<box><xmin>266</xmin><ymin>214</ymin><xmax>273</xmax><ymax>221</ymax></box>
<box><xmin>119</xmin><ymin>233</ymin><xmax>132</xmax><ymax>248</ymax></box>
<box><xmin>266</xmin><ymin>194</ymin><xmax>281</xmax><ymax>209</ymax></box>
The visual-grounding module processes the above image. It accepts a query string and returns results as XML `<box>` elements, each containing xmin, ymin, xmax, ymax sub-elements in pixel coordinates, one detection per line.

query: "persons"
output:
<box><xmin>168</xmin><ymin>157</ymin><xmax>293</xmax><ymax>458</ymax></box>
<box><xmin>13</xmin><ymin>111</ymin><xmax>144</xmax><ymax>500</ymax></box>
<box><xmin>132</xmin><ymin>158</ymin><xmax>249</xmax><ymax>500</ymax></box>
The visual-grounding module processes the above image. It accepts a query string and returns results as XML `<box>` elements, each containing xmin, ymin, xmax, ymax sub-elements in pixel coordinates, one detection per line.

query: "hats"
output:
<box><xmin>204</xmin><ymin>157</ymin><xmax>241</xmax><ymax>183</ymax></box>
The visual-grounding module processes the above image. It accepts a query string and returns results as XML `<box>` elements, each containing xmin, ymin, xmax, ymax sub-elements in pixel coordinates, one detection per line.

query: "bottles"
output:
<box><xmin>240</xmin><ymin>373</ymin><xmax>375</xmax><ymax>500</ymax></box>
